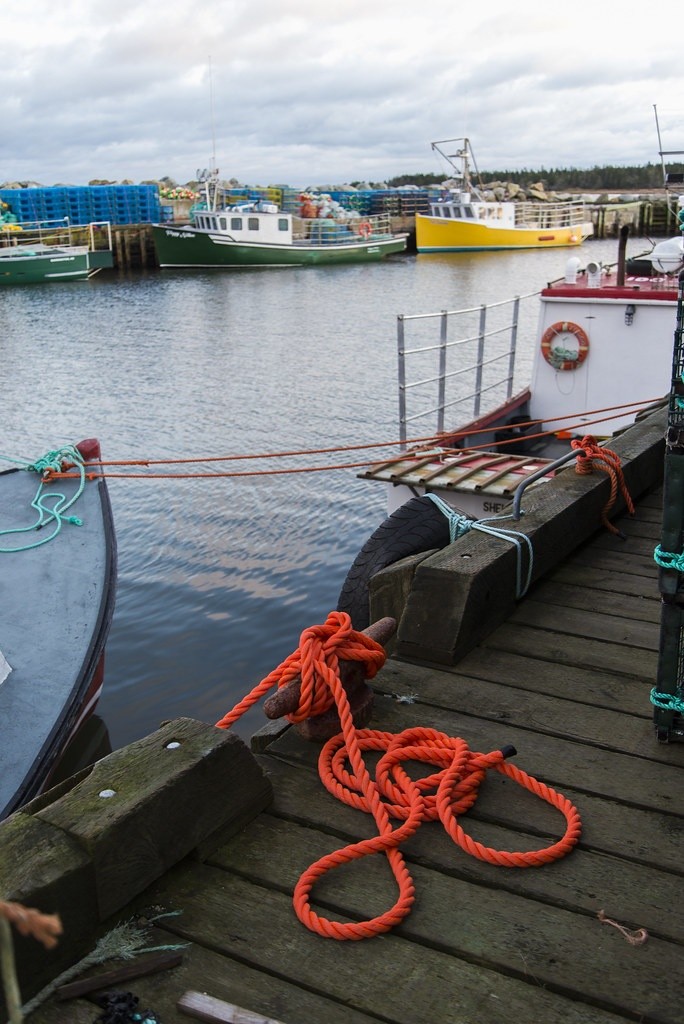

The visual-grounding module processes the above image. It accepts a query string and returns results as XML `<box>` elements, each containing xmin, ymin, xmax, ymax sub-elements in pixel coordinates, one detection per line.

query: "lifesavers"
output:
<box><xmin>540</xmin><ymin>321</ymin><xmax>588</xmax><ymax>371</ymax></box>
<box><xmin>336</xmin><ymin>493</ymin><xmax>483</xmax><ymax>630</ymax></box>
<box><xmin>359</xmin><ymin>222</ymin><xmax>372</xmax><ymax>237</ymax></box>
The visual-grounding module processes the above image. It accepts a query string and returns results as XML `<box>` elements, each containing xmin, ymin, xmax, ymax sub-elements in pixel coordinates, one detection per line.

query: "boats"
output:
<box><xmin>0</xmin><ymin>215</ymin><xmax>116</xmax><ymax>284</ymax></box>
<box><xmin>149</xmin><ymin>166</ymin><xmax>411</xmax><ymax>270</ymax></box>
<box><xmin>413</xmin><ymin>137</ymin><xmax>594</xmax><ymax>253</ymax></box>
<box><xmin>354</xmin><ymin>228</ymin><xmax>684</xmax><ymax>525</ymax></box>
<box><xmin>1</xmin><ymin>436</ymin><xmax>120</xmax><ymax>820</ymax></box>
